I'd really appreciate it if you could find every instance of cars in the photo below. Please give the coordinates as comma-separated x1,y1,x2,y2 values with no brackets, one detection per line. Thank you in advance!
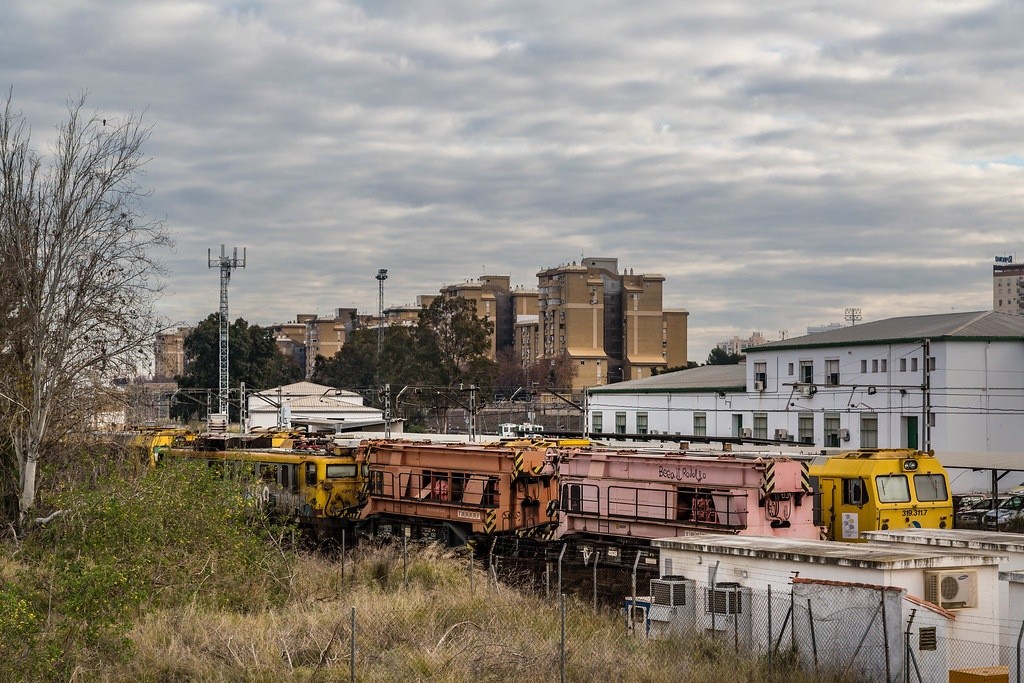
952,481,1024,532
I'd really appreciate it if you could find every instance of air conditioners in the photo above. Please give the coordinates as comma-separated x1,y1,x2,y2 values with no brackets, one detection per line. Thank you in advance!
801,383,810,395
754,381,764,391
837,428,847,439
739,427,753,438
774,429,787,439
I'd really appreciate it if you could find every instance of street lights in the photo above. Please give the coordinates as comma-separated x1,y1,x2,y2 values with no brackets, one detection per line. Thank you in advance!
395,386,409,410
509,387,523,423
374,268,390,384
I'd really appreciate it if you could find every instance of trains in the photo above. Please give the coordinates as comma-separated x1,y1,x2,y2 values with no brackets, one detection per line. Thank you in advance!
43,423,956,542
